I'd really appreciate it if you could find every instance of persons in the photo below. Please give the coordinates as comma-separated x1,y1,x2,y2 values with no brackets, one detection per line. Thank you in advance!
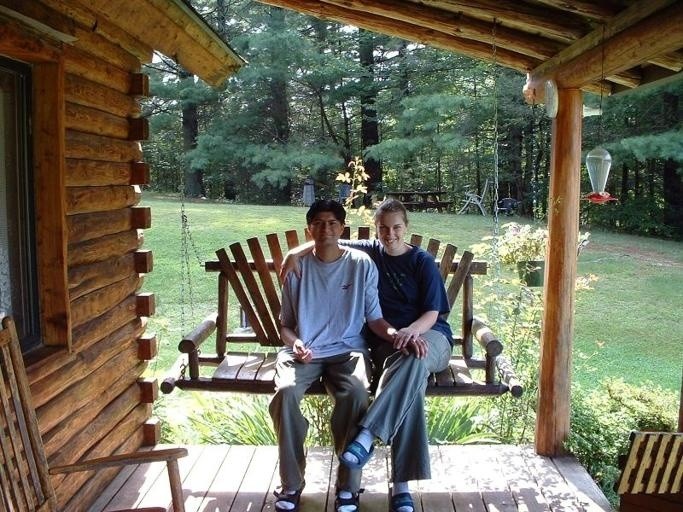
276,197,455,512
268,194,430,512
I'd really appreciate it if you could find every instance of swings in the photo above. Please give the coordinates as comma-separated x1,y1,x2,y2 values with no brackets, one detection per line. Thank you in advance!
161,68,538,396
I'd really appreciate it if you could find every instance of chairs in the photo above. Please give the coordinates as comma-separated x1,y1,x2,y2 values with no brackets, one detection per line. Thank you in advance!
0,316,188,512
457,179,490,217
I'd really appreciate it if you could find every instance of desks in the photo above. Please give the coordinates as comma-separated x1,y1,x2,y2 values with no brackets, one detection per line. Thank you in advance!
385,192,415,212
415,191,448,213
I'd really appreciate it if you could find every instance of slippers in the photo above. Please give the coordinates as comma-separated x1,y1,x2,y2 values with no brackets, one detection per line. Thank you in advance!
339,439,374,469
390,487,415,512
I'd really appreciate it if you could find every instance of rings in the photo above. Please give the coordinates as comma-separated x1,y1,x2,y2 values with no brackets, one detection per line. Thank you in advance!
419,340,424,342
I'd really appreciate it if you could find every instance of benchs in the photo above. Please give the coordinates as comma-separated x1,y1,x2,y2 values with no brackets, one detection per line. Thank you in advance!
159,226,524,398
384,201,454,208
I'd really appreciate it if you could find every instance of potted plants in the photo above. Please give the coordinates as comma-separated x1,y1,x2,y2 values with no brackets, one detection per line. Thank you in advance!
468,221,591,288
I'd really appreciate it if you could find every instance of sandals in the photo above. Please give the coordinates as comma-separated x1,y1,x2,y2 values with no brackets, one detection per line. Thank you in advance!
334,488,364,512
273,480,306,511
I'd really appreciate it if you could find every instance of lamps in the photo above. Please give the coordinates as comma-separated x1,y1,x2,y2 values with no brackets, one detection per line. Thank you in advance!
580,142,619,205
